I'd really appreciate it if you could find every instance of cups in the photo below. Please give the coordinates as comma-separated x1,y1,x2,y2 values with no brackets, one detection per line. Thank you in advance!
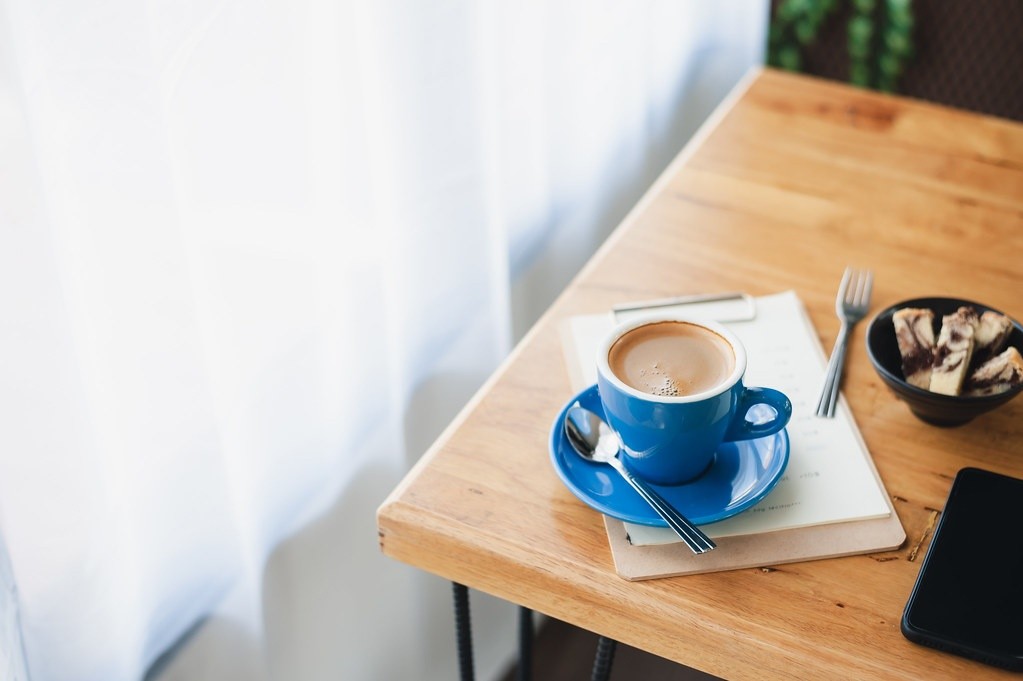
596,314,791,485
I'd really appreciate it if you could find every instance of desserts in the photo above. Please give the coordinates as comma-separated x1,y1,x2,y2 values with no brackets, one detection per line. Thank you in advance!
891,304,1023,397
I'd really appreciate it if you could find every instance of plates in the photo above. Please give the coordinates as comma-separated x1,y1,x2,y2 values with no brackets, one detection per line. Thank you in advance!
548,383,789,528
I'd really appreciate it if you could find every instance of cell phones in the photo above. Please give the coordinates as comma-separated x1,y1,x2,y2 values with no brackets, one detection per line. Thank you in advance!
901,466,1022,672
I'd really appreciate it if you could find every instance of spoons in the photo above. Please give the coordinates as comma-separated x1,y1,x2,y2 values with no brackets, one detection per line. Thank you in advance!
566,408,716,552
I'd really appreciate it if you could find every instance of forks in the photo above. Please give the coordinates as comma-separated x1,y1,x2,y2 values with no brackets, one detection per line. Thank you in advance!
815,269,874,417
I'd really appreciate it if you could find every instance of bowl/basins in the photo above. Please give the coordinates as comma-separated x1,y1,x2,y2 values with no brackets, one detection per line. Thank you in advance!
865,298,1023,429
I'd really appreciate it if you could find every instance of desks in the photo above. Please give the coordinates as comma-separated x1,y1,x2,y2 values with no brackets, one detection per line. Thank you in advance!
374,67,1022,681
